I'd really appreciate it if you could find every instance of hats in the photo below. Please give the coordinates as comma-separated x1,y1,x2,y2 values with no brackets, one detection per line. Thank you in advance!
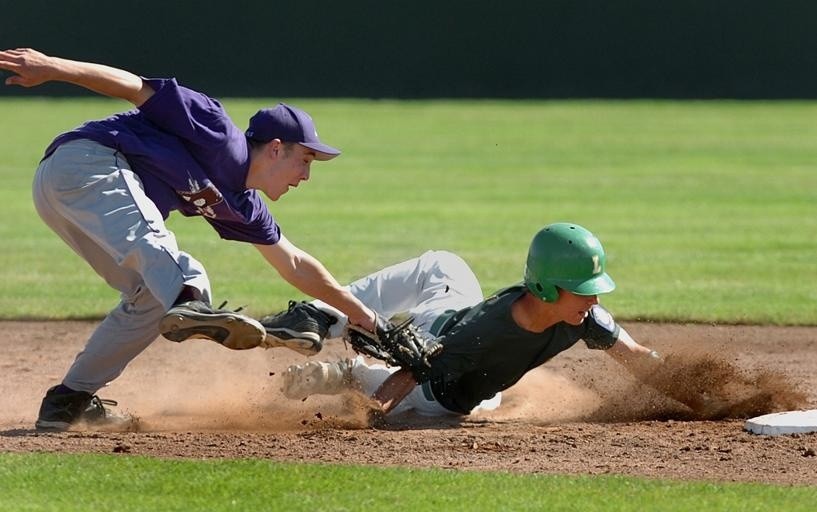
246,103,341,161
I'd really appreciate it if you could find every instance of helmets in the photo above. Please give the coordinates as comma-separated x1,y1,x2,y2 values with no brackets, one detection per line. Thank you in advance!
524,223,616,303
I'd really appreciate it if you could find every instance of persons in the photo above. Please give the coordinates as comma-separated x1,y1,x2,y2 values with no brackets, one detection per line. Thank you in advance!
0,45,443,434
254,218,743,436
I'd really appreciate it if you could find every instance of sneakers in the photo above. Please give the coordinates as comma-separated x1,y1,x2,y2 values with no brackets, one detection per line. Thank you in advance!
159,300,343,399
35,385,121,431
